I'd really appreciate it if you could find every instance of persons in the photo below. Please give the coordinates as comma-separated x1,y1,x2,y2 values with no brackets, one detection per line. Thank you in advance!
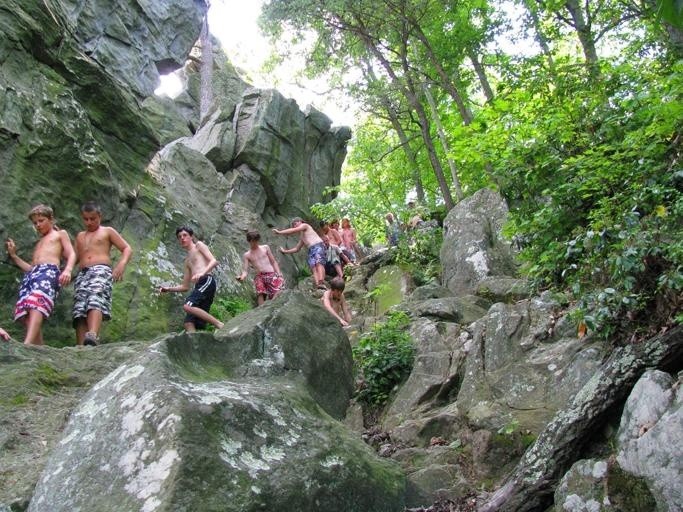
158,226,228,336
321,234,354,278
405,202,430,229
271,216,326,284
339,219,355,250
330,218,350,249
382,213,403,246
70,201,134,348
320,277,352,327
318,220,341,247
4,204,77,342
234,231,284,308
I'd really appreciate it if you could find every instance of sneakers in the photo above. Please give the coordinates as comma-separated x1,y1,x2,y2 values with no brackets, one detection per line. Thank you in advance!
317,280,327,290
83,331,100,346
